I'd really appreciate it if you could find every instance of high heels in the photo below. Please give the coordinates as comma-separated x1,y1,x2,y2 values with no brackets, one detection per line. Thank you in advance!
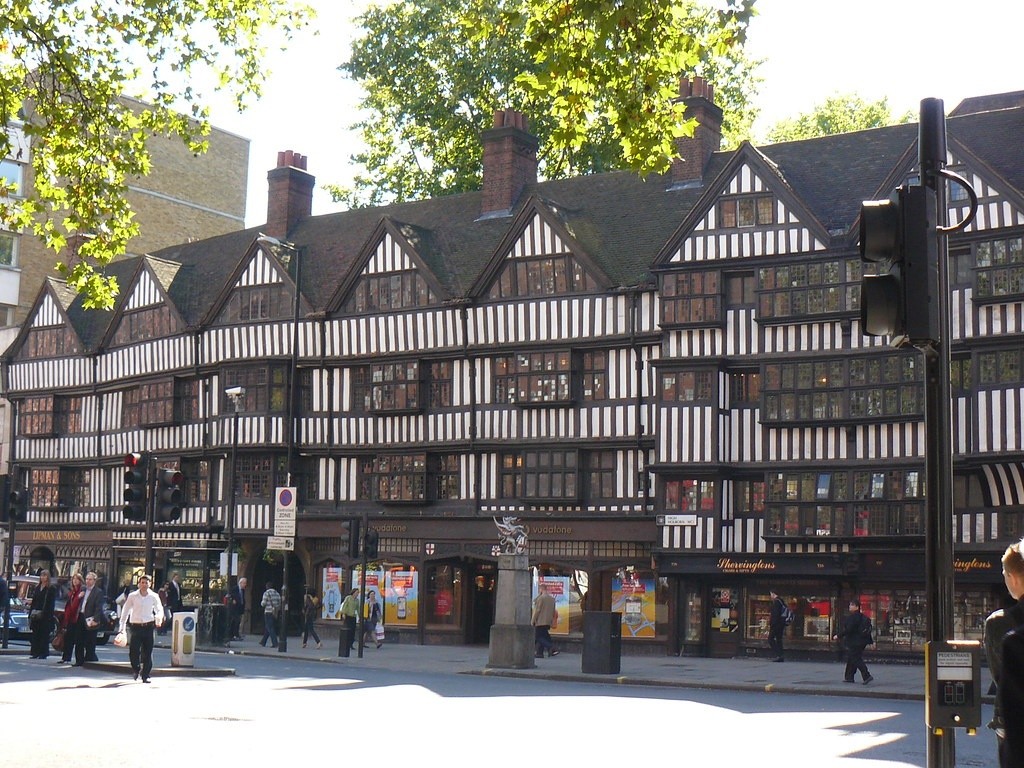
57,659,70,664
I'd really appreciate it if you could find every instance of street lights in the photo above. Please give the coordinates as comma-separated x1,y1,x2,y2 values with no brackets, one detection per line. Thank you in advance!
254,235,302,652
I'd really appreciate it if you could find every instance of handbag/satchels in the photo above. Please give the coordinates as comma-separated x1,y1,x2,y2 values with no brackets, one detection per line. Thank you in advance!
51,626,67,651
85,616,100,627
375,621,386,639
272,606,277,620
335,610,342,620
114,631,128,647
28,609,44,621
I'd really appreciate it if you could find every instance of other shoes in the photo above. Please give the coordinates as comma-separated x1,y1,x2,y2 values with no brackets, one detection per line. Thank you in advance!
362,645,369,648
134,671,139,681
862,675,873,685
376,644,382,649
303,643,307,648
316,641,321,649
72,663,82,667
142,678,151,683
842,679,854,682
30,655,48,659
351,645,356,650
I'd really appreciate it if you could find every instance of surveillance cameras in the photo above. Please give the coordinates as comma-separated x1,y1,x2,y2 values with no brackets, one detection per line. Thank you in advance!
225,386,246,396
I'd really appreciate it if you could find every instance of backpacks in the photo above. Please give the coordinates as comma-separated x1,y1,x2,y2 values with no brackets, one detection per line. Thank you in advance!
775,596,795,626
854,612,872,639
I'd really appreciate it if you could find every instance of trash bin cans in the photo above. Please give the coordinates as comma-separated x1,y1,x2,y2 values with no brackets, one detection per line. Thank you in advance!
339,628,352,658
581,610,624,673
172,611,197,667
200,603,227,644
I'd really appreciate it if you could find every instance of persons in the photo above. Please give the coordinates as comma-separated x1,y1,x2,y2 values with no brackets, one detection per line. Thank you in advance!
767,589,785,662
334,588,383,650
833,599,873,685
259,582,282,649
0,569,183,667
531,584,560,658
984,539,1024,768
302,587,323,649
224,578,249,640
114,575,164,683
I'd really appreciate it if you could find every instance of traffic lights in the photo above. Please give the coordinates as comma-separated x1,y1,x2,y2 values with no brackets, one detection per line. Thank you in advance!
154,469,184,525
123,451,150,522
10,486,27,522
365,531,379,559
860,182,942,347
342,520,359,559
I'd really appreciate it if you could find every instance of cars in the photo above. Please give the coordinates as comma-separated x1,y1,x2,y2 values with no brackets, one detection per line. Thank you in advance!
1,574,120,644
